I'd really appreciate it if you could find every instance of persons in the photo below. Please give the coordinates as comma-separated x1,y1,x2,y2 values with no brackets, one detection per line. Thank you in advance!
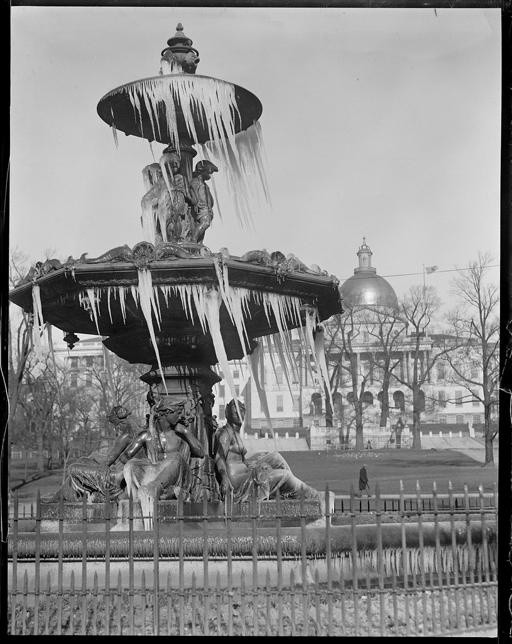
211,396,326,499
122,396,205,501
358,463,371,497
137,152,220,245
67,405,141,501
